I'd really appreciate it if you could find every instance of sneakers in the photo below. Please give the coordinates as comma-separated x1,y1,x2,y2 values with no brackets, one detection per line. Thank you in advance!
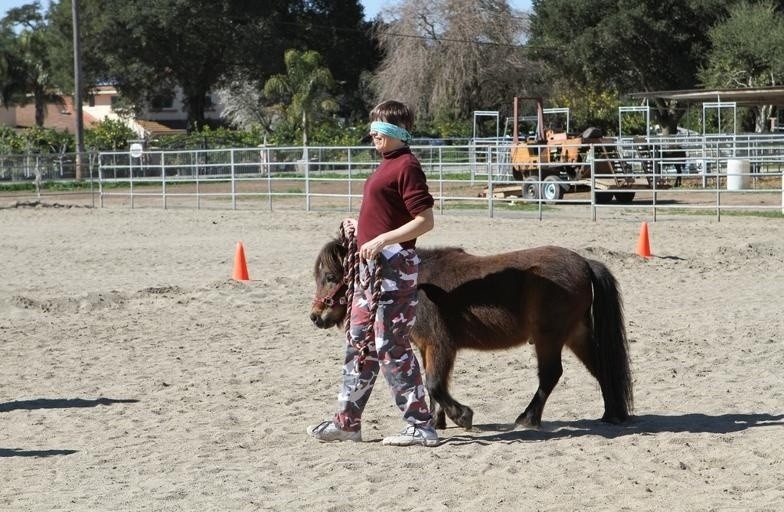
307,422,361,442
382,425,439,446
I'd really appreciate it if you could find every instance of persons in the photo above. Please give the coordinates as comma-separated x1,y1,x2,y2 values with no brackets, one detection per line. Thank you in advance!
307,97,442,447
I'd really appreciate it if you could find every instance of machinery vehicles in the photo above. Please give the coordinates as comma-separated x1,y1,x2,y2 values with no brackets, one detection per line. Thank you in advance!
476,96,671,204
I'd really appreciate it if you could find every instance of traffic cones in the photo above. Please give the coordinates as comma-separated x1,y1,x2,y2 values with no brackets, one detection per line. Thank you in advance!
636,221,650,257
233,241,249,280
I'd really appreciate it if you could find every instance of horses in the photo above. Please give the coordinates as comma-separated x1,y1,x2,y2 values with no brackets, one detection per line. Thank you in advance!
309,232,635,433
651,144,686,187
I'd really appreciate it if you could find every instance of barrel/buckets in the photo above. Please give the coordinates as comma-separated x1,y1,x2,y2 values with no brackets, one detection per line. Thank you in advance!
726,158,751,192
